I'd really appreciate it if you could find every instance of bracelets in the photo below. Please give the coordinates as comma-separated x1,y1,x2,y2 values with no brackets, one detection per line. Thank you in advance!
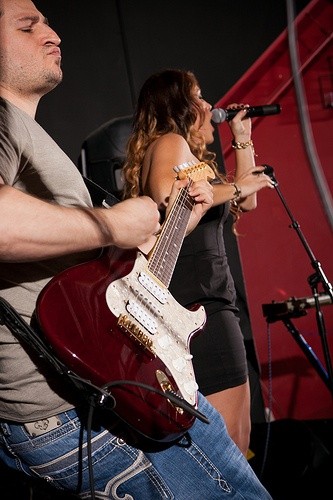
231,140,253,150
231,183,242,198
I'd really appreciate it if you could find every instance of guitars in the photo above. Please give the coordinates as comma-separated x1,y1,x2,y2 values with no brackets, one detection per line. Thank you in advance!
27,158,219,452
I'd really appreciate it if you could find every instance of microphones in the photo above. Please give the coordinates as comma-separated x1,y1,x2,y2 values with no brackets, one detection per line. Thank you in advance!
211,104,282,123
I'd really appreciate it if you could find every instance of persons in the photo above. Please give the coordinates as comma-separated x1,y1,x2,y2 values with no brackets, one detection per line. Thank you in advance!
122,70,278,459
0,0,277,500
